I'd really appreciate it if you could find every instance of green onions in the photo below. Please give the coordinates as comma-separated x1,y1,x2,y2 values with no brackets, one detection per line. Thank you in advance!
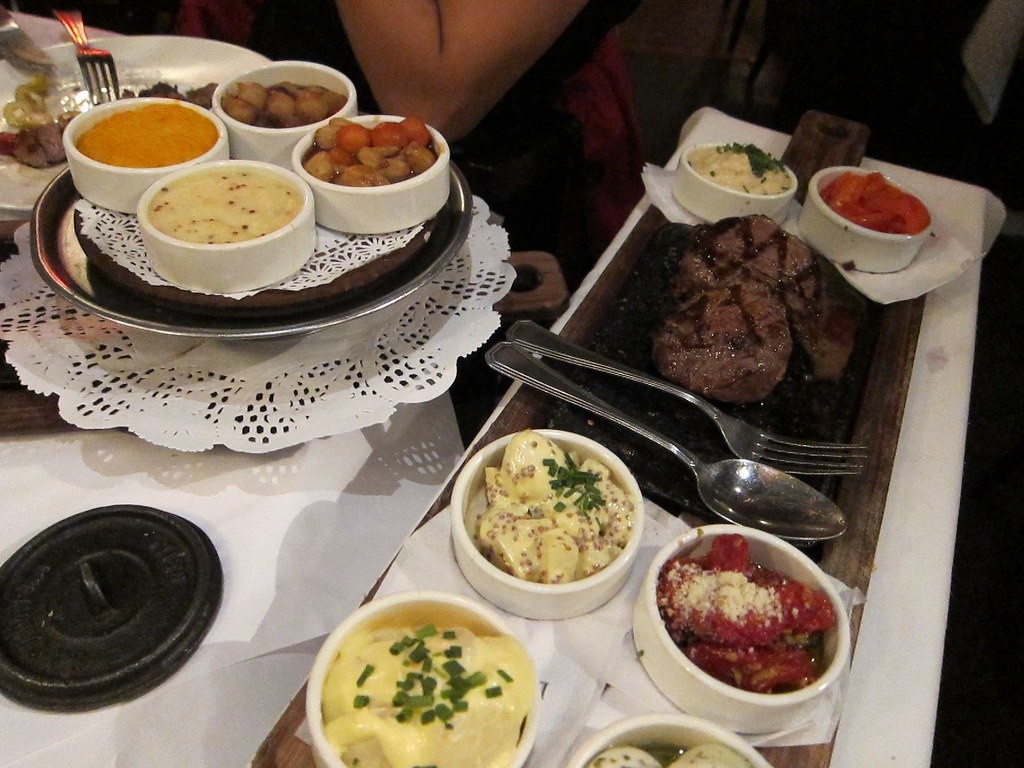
353,624,511,730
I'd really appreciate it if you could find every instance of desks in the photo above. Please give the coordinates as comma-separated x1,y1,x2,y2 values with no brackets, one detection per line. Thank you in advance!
0,10,467,767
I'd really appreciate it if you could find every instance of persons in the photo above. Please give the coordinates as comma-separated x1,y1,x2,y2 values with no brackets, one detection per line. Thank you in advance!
766,1,1024,230
245,1,651,290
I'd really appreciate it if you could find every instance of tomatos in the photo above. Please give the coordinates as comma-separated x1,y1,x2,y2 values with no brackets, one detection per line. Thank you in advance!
821,171,930,235
663,535,835,693
335,117,429,152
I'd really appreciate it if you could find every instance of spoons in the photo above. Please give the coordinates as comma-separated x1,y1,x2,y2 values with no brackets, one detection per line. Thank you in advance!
484,338,847,541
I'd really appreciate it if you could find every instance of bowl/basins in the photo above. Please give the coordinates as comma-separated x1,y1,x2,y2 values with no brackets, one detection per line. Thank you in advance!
564,715,777,766
306,593,540,768
451,427,645,621
211,60,358,165
290,113,451,233
671,141,799,228
799,158,934,271
61,97,231,214
633,523,853,733
136,159,316,295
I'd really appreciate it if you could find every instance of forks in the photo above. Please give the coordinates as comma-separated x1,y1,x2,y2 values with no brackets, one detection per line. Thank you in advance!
51,9,123,103
504,319,871,491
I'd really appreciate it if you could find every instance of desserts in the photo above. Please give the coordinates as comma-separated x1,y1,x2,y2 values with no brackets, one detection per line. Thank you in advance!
146,167,304,245
74,102,219,168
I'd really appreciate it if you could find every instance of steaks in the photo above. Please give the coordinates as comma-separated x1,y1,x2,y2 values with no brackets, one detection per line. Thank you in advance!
648,214,866,405
9,80,220,168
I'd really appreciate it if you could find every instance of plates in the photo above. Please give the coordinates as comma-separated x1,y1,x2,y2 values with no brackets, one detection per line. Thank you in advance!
0,34,297,213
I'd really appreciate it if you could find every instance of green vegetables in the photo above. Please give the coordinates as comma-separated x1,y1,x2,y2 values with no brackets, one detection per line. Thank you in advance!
710,143,790,195
543,453,606,520
